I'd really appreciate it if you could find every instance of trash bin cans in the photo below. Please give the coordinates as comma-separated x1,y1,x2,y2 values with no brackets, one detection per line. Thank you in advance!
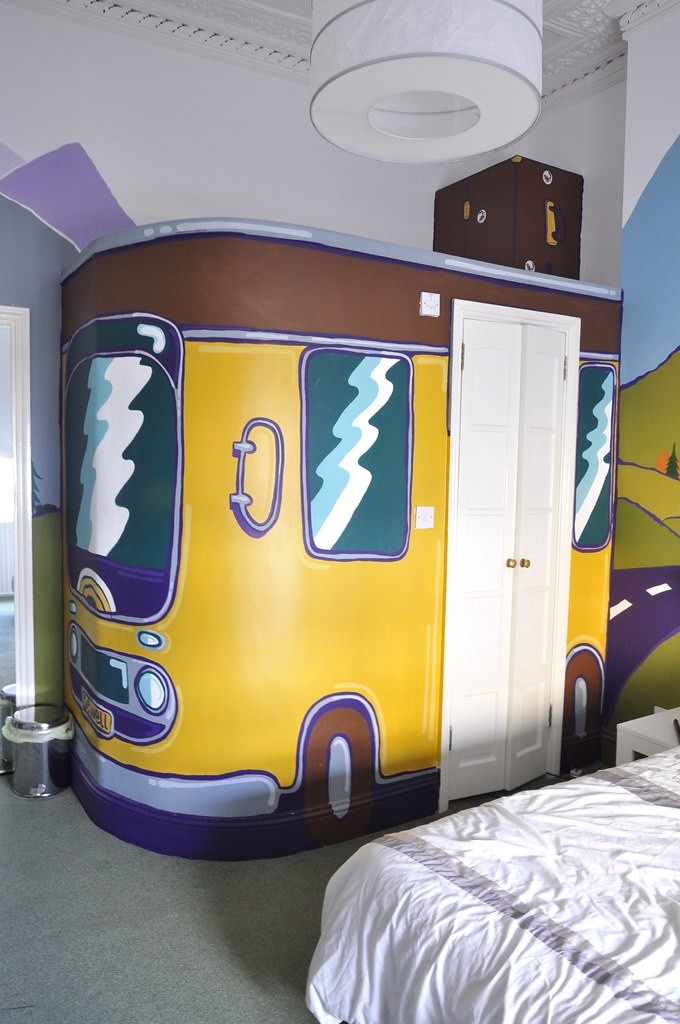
1,705,77,799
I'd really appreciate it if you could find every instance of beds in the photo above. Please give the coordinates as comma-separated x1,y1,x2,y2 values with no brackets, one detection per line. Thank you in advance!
307,747,680,1024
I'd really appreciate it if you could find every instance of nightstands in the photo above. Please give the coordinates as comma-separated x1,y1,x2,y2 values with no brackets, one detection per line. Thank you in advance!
617,707,680,767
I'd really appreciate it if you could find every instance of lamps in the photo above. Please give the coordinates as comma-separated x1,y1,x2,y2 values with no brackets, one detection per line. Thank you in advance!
308,0,543,164
